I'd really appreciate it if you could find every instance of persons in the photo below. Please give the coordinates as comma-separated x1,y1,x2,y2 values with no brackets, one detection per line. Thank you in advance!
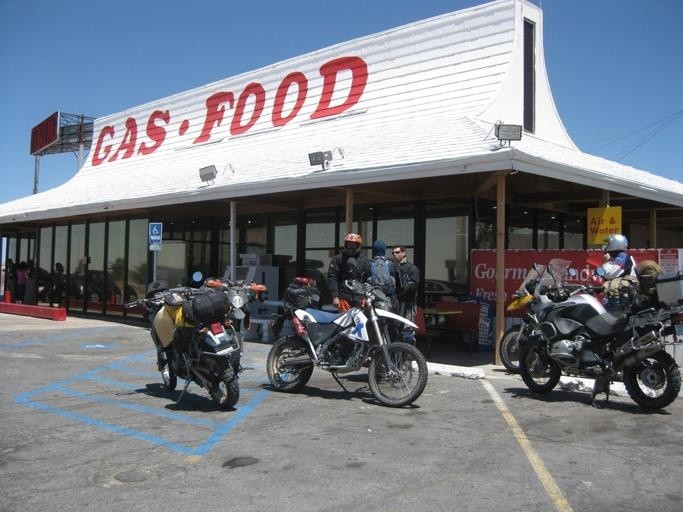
327,233,368,315
595,233,638,312
1,258,16,303
15,262,27,304
368,239,404,377
389,244,421,368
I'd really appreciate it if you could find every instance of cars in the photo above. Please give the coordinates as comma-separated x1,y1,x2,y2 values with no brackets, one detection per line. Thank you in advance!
38,269,147,306
422,277,469,303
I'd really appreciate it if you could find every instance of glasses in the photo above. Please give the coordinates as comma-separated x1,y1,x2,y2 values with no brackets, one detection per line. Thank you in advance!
392,251,401,255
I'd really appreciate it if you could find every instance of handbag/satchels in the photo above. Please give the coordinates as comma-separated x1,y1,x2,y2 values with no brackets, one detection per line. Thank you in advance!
182,290,230,326
601,275,639,307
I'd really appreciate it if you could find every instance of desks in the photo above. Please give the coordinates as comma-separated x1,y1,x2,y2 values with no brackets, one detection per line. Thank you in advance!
263,299,294,309
421,310,463,331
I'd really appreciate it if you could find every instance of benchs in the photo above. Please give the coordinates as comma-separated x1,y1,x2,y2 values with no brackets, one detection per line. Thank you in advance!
428,301,481,353
246,319,275,343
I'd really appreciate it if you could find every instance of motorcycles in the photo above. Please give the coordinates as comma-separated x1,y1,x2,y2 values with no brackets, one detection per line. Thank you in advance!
266,277,429,408
124,270,268,410
498,257,682,410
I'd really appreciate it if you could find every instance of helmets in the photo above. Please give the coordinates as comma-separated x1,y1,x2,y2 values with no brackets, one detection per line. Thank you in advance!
344,234,362,255
603,234,628,253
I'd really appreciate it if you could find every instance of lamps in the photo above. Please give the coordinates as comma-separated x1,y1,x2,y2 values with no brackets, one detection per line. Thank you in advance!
492,122,523,146
199,165,220,184
307,152,336,171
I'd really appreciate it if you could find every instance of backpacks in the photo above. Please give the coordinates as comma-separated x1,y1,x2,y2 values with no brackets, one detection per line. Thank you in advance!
369,258,395,295
